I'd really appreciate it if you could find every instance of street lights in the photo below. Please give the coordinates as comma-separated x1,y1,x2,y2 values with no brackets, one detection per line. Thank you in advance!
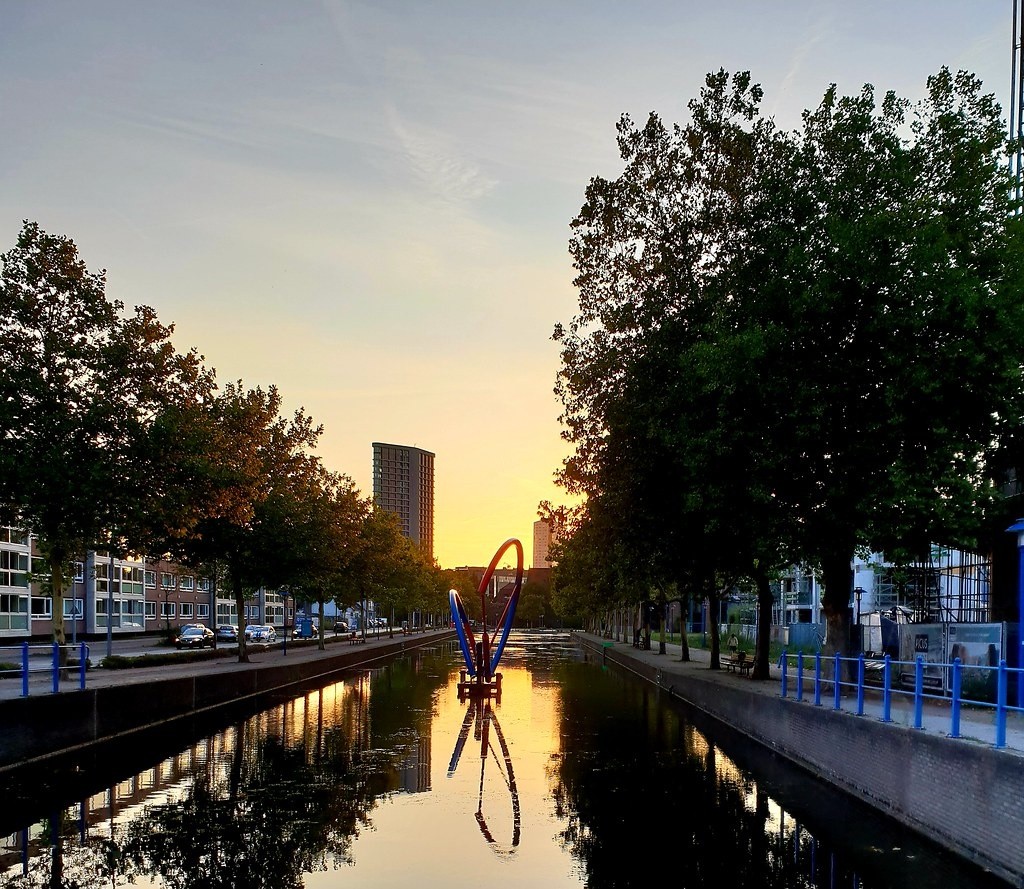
701,601,709,648
670,604,675,641
752,597,761,650
1005,519,1024,711
850,586,868,689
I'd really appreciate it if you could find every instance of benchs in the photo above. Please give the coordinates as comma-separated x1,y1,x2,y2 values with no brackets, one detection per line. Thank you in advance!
349,639,364,645
634,638,647,649
720,653,756,676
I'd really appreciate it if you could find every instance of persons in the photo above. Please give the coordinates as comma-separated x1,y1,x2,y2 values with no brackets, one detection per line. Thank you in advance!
312,621,319,638
635,628,642,648
726,633,738,653
403,625,406,636
382,621,384,629
352,632,358,644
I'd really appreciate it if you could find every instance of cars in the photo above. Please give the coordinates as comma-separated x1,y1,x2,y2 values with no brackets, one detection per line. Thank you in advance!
171,622,205,643
216,625,239,642
333,621,349,633
175,627,215,649
245,624,261,640
292,624,319,638
252,625,277,642
